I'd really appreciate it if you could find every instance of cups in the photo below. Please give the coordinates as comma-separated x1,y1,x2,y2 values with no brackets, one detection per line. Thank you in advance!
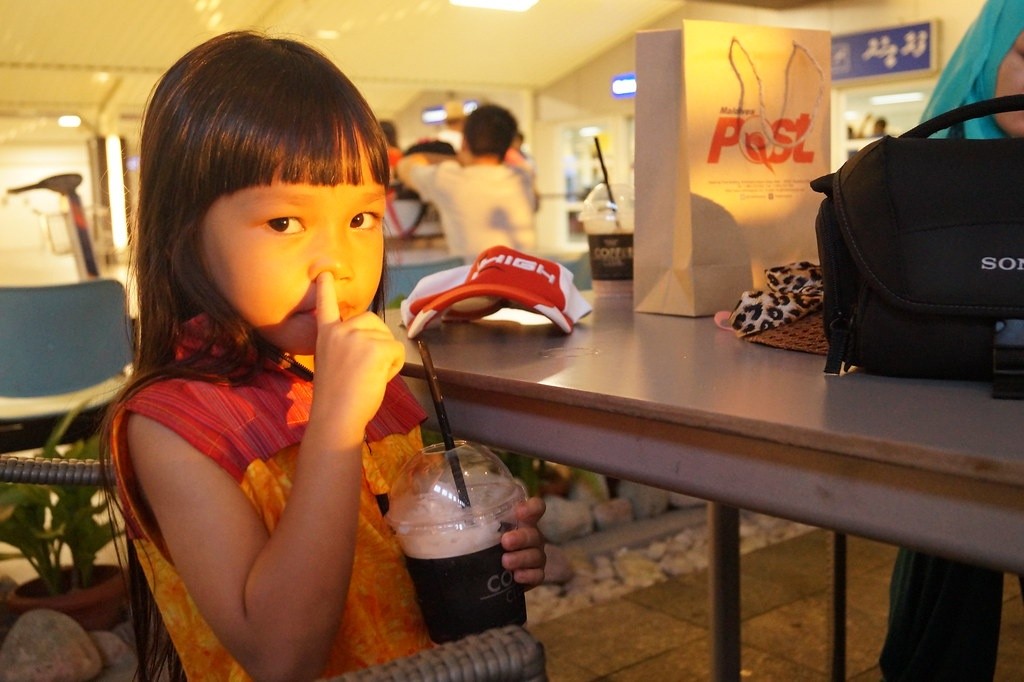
577,178,634,298
382,440,529,648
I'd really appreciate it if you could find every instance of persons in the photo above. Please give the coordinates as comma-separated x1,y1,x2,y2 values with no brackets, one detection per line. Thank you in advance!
104,31,550,682
376,104,540,257
878,0,1024,682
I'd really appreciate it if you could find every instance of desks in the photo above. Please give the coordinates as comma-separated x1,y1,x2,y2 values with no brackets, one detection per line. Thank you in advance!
369,281,1024,682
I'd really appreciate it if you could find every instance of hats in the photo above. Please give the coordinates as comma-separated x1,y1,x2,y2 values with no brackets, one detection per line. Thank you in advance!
730,261,830,356
402,244,592,339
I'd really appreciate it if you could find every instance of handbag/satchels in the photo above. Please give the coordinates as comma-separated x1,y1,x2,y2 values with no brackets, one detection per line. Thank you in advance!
635,20,831,317
810,93,1024,400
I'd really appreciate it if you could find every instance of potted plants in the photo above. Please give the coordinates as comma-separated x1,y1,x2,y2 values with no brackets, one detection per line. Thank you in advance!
0,379,129,631
